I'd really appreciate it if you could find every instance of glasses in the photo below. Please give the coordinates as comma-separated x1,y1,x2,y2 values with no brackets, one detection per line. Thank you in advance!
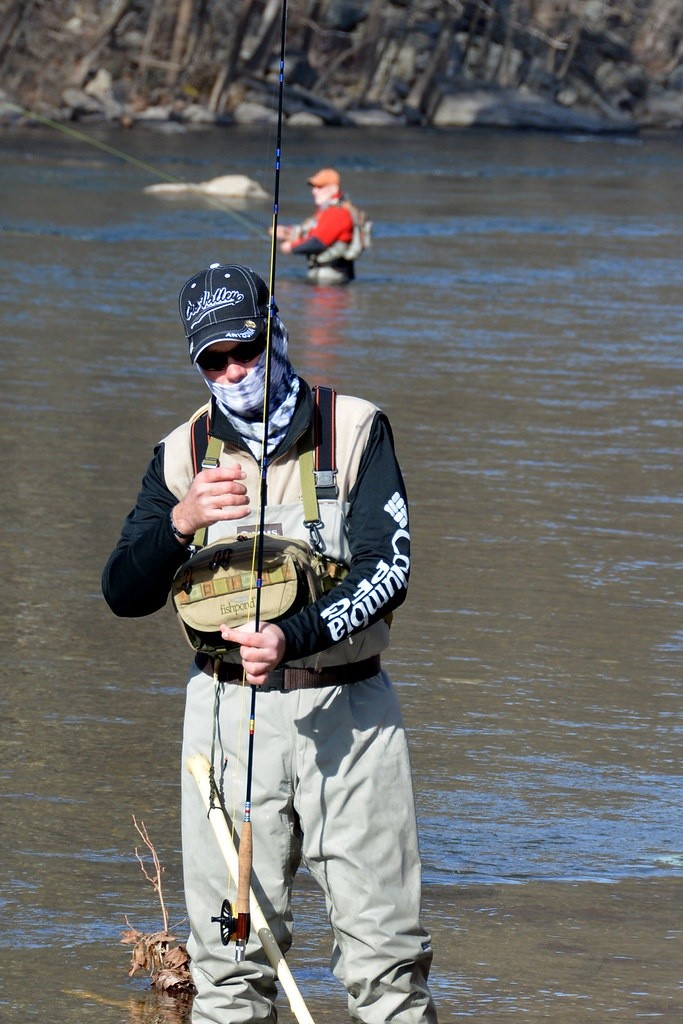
196,332,266,371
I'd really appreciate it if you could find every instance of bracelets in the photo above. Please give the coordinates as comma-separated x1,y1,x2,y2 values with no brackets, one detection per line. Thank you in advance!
170,512,195,539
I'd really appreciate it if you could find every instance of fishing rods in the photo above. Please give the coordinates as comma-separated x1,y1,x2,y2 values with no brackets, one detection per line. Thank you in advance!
0,95,283,242
223,0,291,971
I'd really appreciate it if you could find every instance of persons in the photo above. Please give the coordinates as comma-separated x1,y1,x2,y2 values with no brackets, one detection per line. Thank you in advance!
101,263,439,1024
269,169,355,284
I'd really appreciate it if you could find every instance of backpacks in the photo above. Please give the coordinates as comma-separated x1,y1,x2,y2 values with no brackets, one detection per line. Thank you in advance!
338,200,375,261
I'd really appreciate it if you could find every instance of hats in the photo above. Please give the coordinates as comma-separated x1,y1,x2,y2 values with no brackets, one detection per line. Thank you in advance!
178,263,279,366
307,167,341,187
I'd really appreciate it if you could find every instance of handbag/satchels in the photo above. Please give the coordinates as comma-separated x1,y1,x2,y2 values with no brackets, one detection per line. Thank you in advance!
171,436,331,657
